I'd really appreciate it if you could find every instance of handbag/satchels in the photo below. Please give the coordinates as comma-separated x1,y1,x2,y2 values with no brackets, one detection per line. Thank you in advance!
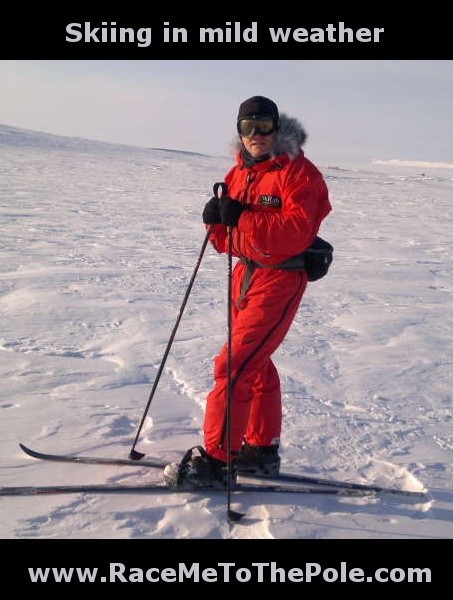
240,236,333,282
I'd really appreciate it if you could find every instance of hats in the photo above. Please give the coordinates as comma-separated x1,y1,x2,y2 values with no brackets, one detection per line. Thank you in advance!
237,96,279,127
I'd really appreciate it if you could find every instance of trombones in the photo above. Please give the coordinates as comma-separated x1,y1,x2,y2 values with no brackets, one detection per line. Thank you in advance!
0,443,422,496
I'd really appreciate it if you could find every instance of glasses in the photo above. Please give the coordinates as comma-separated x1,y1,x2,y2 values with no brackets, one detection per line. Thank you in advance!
237,115,275,136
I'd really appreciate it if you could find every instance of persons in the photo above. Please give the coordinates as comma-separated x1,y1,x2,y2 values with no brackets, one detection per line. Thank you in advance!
163,94,333,491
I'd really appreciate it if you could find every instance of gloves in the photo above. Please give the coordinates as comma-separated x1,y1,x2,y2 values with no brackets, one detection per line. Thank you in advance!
203,197,222,224
219,198,242,226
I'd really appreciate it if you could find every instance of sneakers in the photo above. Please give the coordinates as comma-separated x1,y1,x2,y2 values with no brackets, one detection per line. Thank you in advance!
238,444,280,480
163,445,239,490
